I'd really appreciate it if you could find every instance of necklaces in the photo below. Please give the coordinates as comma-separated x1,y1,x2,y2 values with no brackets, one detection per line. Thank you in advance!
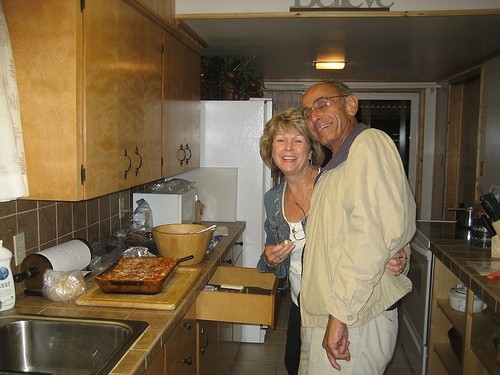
286,166,315,234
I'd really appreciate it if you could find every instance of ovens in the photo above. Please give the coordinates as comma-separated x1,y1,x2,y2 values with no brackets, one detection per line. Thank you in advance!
398,234,433,375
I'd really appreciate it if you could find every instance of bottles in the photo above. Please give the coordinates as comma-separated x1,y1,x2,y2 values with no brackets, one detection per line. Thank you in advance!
127,198,153,231
0,240,15,312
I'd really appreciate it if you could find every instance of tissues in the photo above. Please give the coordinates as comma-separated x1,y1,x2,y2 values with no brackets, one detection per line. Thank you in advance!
491,217,500,259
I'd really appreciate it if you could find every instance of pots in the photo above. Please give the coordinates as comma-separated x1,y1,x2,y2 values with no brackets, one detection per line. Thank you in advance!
447,207,475,228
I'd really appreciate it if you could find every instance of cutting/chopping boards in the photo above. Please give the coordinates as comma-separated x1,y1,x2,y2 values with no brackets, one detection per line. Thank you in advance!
75,267,202,311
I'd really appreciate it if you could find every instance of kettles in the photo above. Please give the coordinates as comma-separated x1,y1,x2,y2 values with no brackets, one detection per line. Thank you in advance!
449,284,488,312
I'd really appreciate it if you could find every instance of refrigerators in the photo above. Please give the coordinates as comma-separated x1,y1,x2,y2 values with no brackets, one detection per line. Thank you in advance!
198,97,275,344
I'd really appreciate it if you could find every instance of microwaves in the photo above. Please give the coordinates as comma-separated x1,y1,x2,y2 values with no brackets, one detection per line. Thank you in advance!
133,187,197,226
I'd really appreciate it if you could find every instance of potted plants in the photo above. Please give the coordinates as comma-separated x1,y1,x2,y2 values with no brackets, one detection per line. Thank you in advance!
218,54,267,100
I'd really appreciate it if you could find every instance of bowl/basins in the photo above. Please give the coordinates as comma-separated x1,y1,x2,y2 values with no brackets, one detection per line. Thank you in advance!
447,327,463,364
151,224,213,266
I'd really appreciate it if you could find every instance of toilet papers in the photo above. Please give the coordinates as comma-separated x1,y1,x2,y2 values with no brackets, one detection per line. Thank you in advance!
20,239,94,292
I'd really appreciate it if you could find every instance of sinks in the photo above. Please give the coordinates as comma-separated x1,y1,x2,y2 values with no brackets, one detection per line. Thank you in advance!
0,310,151,375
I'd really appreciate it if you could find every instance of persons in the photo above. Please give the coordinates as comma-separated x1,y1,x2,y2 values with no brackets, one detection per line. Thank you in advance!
256,106,407,375
296,80,417,375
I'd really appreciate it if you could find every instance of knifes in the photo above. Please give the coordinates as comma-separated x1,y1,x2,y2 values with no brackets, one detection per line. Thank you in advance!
479,192,500,236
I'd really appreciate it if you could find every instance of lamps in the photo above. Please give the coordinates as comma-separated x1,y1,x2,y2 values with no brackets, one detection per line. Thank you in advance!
311,50,351,71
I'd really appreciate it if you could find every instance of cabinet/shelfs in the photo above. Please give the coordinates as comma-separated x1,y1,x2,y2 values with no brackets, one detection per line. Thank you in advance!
136,221,280,375
398,235,500,374
0,0,209,203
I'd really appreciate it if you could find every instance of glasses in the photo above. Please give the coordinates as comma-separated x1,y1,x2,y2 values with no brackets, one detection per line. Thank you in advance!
302,94,349,121
273,201,307,246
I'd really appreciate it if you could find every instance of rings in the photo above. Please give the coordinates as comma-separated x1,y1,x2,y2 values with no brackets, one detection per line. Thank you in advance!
279,257,284,262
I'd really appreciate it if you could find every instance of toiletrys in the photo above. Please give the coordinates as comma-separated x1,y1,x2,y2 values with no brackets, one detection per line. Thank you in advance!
0,239,16,312
